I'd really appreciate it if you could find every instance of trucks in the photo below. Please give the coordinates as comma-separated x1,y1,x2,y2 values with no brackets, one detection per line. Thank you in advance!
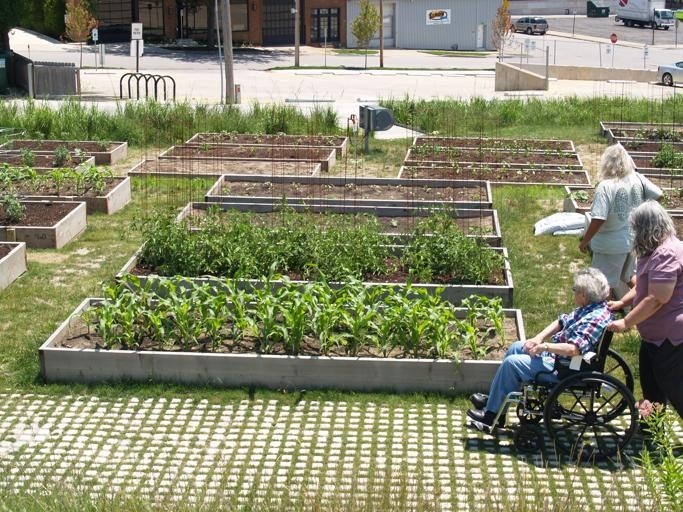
615,0,675,31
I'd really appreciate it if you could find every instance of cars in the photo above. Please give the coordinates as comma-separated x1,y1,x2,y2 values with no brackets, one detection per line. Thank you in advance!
657,60,683,86
510,16,549,35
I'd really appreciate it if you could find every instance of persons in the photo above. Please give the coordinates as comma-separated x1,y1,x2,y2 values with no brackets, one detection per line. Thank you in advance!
462,265,613,427
605,196,683,438
578,141,664,319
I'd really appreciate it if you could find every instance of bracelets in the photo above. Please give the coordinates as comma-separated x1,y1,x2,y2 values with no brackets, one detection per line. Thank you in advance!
542,341,548,354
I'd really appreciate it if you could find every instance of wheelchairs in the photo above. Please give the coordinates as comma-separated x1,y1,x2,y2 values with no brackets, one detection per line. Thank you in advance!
464,308,639,461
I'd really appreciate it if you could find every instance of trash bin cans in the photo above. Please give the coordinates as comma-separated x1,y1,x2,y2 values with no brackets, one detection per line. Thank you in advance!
565,9,569,15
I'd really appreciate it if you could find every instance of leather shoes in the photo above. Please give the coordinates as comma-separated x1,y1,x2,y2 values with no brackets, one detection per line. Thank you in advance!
466,393,506,427
